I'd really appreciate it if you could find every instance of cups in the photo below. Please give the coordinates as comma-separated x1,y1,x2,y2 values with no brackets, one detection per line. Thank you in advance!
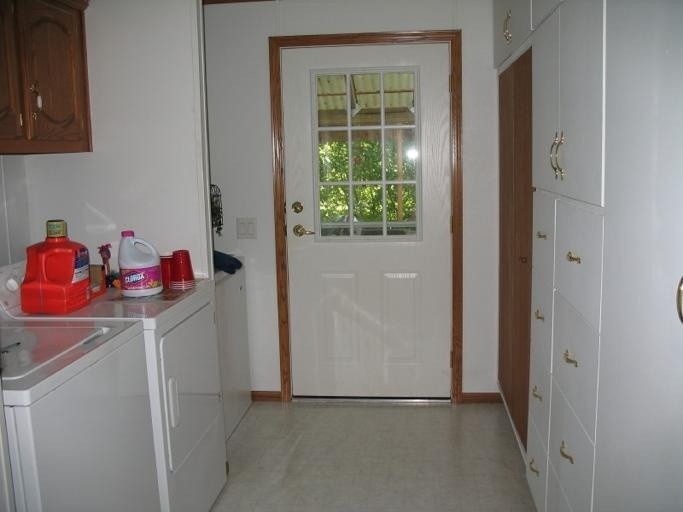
159,249,195,290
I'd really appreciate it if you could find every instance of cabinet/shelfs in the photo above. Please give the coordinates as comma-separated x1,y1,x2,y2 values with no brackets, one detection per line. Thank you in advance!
0,0,91,153
492,1,562,66
531,0,607,208
525,192,605,512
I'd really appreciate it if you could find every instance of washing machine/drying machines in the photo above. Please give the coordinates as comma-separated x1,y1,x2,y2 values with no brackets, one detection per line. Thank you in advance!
0,260,229,512
1,302,161,512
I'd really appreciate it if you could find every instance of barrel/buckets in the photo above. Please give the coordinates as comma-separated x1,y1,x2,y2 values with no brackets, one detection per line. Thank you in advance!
117,232,164,296
19,221,92,314
